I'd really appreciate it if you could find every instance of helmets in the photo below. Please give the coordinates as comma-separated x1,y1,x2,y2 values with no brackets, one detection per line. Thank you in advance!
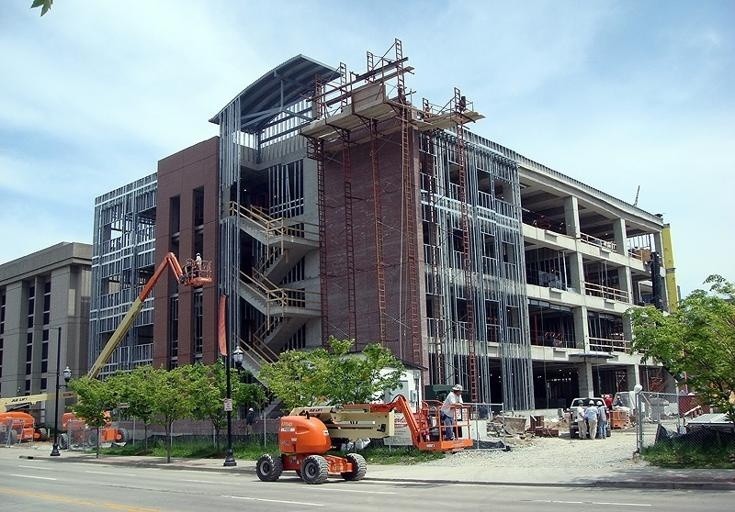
577,399,602,406
452,383,463,391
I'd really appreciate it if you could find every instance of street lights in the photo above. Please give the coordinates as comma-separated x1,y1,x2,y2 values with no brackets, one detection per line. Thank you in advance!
224,294,245,467
49,324,72,456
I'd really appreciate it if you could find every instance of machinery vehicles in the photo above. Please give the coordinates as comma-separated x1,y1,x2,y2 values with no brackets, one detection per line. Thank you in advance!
0,390,78,443
256,393,474,483
59,250,214,450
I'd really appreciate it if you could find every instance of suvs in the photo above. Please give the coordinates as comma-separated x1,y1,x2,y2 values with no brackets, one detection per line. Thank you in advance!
566,397,612,437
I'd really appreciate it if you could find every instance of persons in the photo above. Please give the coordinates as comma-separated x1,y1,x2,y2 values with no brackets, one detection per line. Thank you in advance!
605,395,611,407
585,400,598,439
577,399,587,439
194,252,203,277
246,408,258,435
597,401,608,439
440,383,465,441
613,396,622,406
